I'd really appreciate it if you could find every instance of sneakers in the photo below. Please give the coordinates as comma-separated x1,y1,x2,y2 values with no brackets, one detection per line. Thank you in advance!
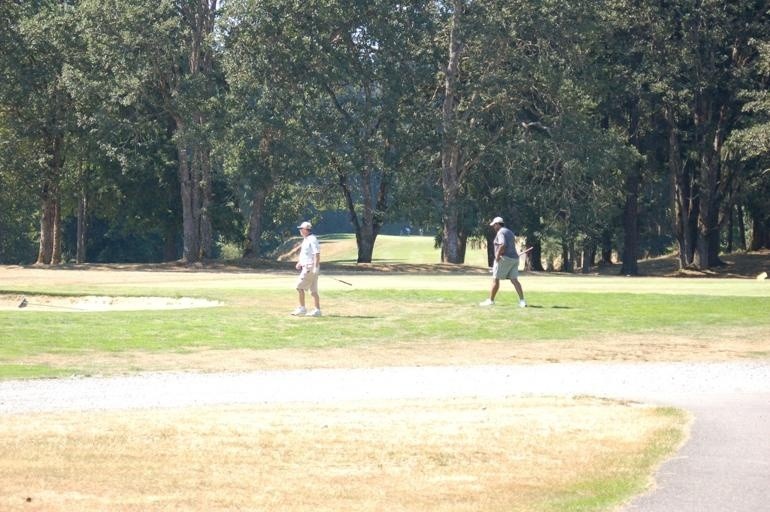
480,298,495,306
305,307,321,317
291,306,306,315
519,299,527,308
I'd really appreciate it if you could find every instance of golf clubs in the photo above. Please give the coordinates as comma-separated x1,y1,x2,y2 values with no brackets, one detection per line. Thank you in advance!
307,265,352,286
489,246,534,273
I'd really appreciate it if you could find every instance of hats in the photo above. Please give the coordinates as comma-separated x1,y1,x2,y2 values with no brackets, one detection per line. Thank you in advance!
489,216,504,227
296,222,312,231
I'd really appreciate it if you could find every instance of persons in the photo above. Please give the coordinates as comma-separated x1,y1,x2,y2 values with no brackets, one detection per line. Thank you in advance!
479,215,528,308
294,220,321,317
